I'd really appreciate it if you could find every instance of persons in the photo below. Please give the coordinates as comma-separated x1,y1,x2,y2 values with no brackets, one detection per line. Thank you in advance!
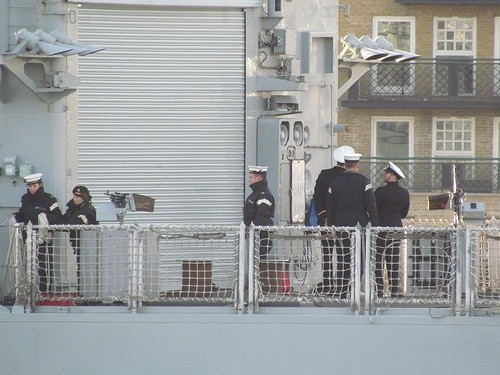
313,145,357,295
243,165,275,260
326,154,380,300
10,172,62,295
47,184,100,296
373,161,410,299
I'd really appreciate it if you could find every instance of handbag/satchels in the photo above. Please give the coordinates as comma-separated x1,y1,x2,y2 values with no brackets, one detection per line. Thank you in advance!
306,199,319,227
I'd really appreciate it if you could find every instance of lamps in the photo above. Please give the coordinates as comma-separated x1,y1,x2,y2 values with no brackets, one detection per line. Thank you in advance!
10,28,106,57
339,33,422,64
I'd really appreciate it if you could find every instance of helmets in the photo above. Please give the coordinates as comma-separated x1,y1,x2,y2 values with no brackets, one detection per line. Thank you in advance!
72,186,92,201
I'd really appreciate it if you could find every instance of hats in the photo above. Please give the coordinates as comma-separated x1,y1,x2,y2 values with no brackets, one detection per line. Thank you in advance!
343,153,362,163
383,161,406,180
23,173,43,185
248,166,269,174
334,146,355,166
